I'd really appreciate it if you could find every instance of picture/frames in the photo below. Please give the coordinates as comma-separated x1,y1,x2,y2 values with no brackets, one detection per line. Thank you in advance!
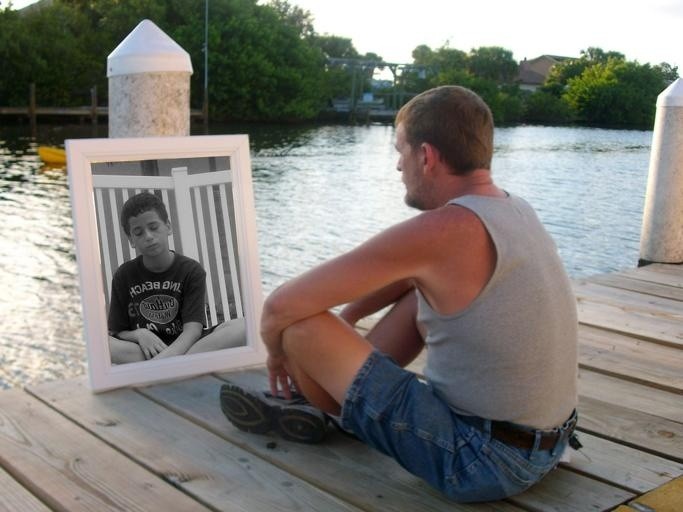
59,132,269,397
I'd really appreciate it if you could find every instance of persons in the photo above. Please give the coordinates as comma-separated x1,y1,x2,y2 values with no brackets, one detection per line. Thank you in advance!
219,85,582,504
108,192,246,365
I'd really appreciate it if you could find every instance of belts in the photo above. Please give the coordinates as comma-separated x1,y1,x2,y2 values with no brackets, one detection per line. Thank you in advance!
453,412,561,453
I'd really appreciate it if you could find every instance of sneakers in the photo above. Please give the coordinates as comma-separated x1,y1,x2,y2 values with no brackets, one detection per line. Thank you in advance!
218,382,337,445
